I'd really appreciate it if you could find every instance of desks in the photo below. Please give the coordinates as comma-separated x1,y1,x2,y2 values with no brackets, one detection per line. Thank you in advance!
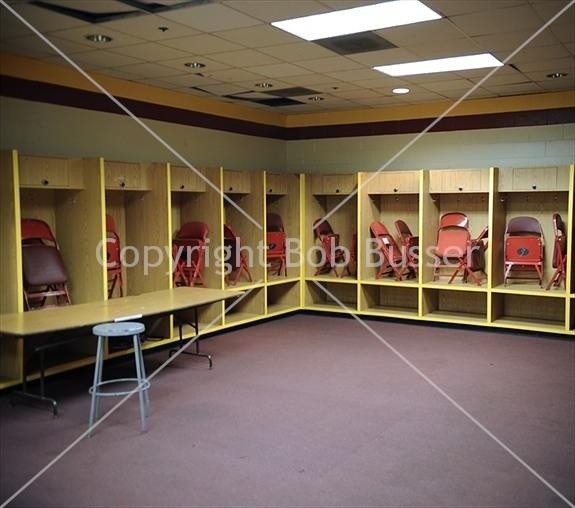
0,286,245,416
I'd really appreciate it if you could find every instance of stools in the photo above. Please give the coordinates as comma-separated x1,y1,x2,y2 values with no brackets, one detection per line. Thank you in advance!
89,323,152,438
